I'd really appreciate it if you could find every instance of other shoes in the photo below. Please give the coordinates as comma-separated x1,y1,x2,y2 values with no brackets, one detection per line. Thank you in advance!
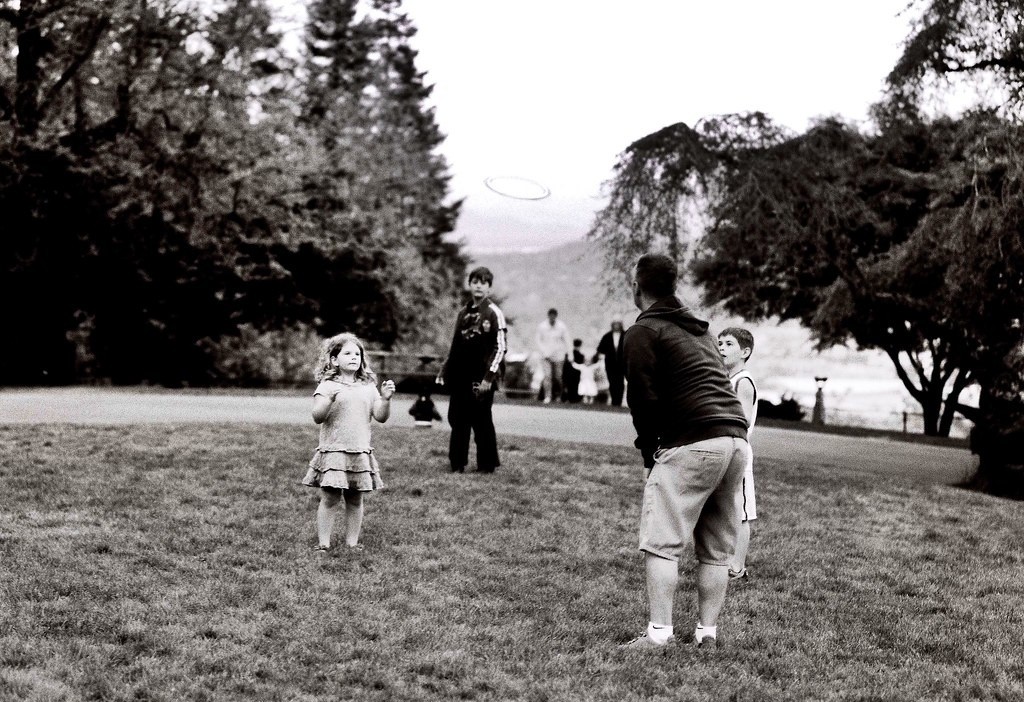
446,463,464,474
727,563,749,582
475,465,495,474
682,630,718,650
617,633,678,654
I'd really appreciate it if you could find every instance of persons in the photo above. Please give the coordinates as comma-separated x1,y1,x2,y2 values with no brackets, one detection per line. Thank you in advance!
435,267,509,473
302,333,396,552
528,308,624,407
616,254,750,651
715,327,758,581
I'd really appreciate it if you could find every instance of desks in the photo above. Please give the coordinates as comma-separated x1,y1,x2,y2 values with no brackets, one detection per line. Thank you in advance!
365,349,441,395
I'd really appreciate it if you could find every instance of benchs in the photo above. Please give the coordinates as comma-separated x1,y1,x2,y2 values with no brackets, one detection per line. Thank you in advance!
371,369,437,381
